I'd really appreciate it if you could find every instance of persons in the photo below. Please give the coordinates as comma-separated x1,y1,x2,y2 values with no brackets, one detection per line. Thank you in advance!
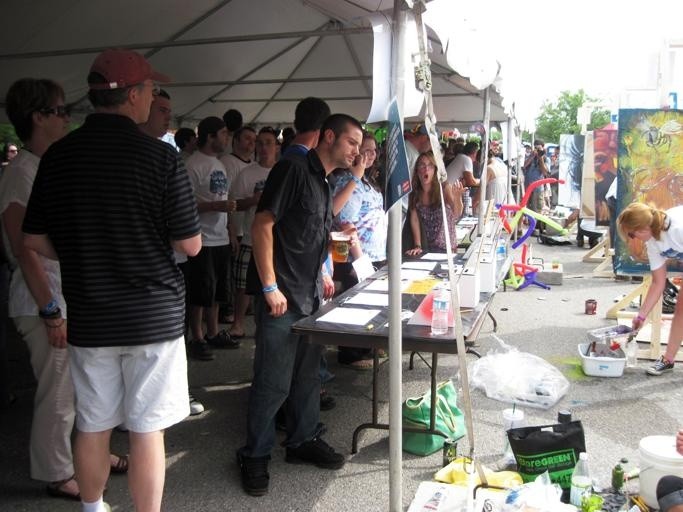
174,127,198,160
0,141,20,168
222,109,243,133
218,125,257,187
400,124,442,230
233,113,365,498
224,126,281,339
404,151,469,256
333,134,389,263
470,138,520,216
656,431,683,512
138,87,173,138
615,202,683,377
21,48,207,511
276,97,368,438
443,138,480,186
1,77,130,502
182,115,263,362
520,138,559,213
378,139,387,193
564,209,609,248
279,126,295,162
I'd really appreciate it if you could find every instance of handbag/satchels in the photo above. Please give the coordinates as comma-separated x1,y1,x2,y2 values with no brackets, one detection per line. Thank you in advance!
401,379,465,457
506,412,587,506
537,234,571,245
661,278,679,313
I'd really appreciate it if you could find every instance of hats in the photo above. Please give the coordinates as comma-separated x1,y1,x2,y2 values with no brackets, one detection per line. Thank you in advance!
260,127,281,137
417,125,440,137
88,50,171,90
491,142,499,146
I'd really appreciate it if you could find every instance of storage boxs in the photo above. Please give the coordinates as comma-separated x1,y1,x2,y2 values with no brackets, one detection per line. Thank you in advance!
578,342,625,377
588,324,635,348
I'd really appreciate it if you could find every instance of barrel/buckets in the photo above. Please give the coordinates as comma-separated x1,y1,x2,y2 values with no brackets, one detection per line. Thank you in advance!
637,433,683,509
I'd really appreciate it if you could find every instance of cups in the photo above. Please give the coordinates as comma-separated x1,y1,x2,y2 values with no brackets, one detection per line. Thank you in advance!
581,494,604,512
501,408,525,456
330,231,349,263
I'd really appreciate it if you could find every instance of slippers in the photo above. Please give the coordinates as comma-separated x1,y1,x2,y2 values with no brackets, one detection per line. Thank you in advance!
364,346,388,358
341,360,372,370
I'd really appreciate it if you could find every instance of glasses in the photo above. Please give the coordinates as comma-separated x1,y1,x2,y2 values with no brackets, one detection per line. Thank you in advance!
628,228,638,239
136,80,161,96
415,162,434,171
41,105,72,119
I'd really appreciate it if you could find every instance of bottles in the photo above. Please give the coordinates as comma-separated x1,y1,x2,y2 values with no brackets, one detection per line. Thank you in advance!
495,240,507,270
569,452,592,508
248,344,255,380
431,285,450,334
627,338,638,368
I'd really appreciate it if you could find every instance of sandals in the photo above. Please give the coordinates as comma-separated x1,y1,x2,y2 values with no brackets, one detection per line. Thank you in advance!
110,450,130,473
45,475,108,500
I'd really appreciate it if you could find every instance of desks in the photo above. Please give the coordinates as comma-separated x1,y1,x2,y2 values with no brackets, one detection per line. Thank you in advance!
290,251,512,455
438,212,522,333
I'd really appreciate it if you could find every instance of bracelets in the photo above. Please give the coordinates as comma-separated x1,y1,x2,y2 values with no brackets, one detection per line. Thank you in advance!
236,197,244,212
351,175,359,184
262,284,279,293
43,317,65,329
637,315,646,322
414,244,422,248
38,298,62,319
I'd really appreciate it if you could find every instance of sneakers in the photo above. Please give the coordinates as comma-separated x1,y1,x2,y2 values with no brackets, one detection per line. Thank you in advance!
590,242,597,248
189,389,205,415
646,355,674,376
320,391,337,411
577,239,584,246
285,436,345,471
221,311,235,324
275,421,327,438
237,447,270,496
189,339,215,360
203,329,239,349
